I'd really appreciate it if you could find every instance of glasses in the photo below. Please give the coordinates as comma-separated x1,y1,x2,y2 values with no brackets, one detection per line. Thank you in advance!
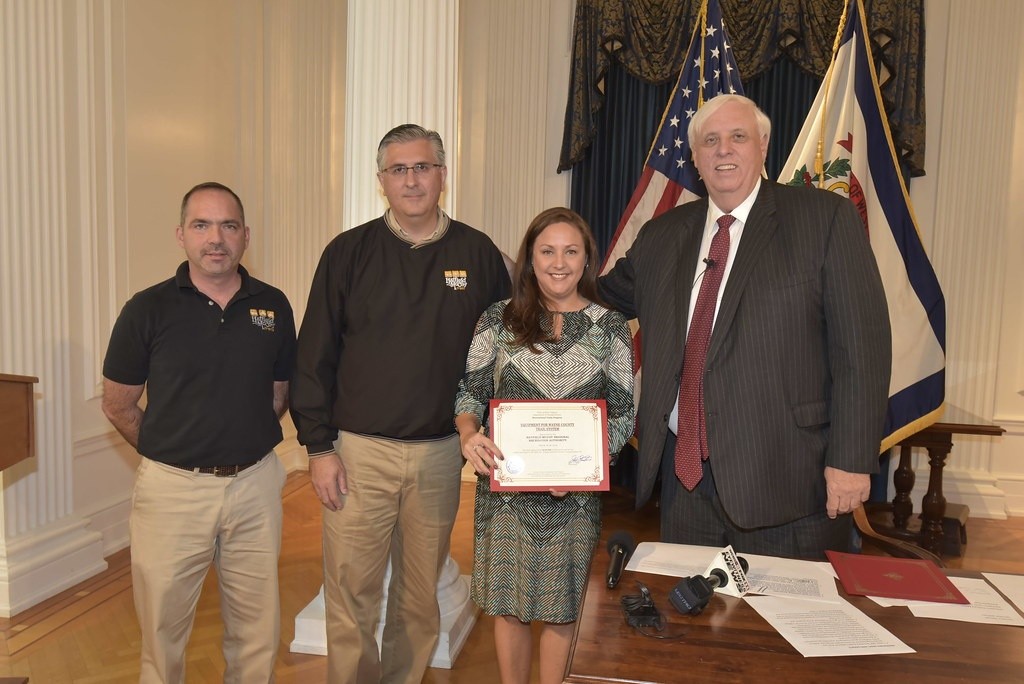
381,162,442,176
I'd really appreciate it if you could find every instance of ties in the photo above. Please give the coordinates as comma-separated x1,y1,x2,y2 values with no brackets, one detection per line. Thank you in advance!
675,215,738,491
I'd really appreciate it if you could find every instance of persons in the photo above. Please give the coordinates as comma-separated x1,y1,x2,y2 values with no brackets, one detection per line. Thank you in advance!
101,181,296,684
501,94,892,560
454,207,635,684
287,123,513,684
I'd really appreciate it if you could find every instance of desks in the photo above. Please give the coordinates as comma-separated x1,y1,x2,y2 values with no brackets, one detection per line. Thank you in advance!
561,539,1024,684
870,400,1004,552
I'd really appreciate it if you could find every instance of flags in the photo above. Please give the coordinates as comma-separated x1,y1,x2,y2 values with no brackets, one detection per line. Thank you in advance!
596,0,770,452
777,0,946,458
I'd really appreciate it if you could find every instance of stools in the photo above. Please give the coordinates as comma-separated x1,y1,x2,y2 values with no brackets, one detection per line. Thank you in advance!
918,504,969,557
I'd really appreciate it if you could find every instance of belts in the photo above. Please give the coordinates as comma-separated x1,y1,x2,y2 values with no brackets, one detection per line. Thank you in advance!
164,456,256,477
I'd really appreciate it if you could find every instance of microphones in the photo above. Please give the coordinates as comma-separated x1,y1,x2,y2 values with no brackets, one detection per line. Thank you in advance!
606,532,634,589
668,545,749,616
706,259,714,269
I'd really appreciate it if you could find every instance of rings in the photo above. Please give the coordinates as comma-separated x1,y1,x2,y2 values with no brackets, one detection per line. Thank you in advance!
473,444,482,451
